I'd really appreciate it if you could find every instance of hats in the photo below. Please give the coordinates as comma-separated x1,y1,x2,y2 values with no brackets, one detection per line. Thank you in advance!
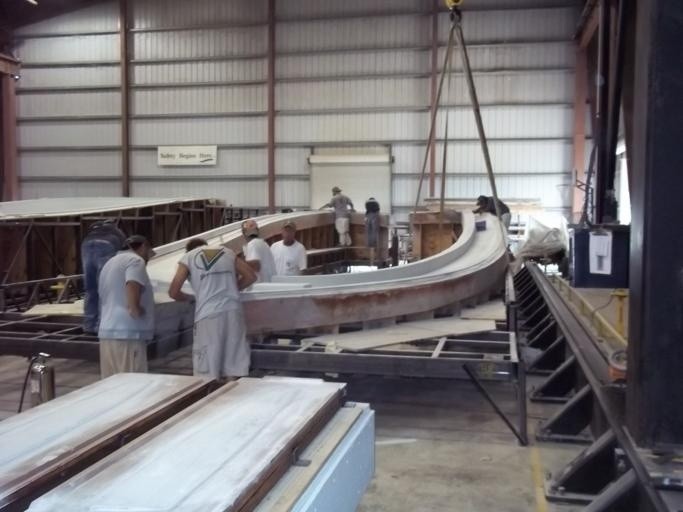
241,220,258,237
476,195,487,206
283,220,296,229
122,236,156,258
333,187,341,192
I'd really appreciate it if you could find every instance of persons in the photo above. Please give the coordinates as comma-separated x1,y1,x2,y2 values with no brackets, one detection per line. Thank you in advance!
168,238,257,381
473,196,512,231
365,195,380,245
97,235,157,379
326,185,354,246
270,221,308,345
240,220,275,283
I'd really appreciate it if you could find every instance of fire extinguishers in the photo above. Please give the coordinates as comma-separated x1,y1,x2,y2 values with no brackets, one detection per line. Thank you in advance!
18,353,54,413
